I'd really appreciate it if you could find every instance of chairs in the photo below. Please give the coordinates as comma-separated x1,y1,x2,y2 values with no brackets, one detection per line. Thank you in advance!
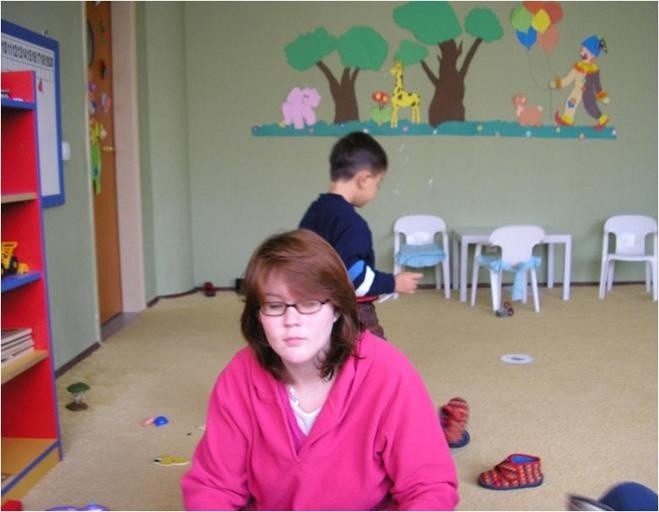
392,214,452,299
470,224,540,313
598,213,657,302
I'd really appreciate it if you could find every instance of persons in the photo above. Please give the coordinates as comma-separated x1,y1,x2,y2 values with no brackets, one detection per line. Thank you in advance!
296,130,424,342
178,226,461,511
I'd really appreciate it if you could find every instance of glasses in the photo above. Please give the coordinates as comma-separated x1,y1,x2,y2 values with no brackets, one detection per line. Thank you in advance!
260,299,329,316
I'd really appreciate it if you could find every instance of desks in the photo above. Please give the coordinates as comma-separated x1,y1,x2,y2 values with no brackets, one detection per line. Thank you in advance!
452,228,573,304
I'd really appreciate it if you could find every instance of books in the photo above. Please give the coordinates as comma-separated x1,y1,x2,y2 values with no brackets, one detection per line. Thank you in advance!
1,327,35,368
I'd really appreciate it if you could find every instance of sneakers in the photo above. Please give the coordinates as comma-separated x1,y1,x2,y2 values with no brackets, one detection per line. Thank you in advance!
440,398,469,447
479,454,542,490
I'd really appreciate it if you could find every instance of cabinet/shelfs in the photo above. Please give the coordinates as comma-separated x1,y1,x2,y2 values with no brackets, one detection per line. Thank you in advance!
1,68,64,507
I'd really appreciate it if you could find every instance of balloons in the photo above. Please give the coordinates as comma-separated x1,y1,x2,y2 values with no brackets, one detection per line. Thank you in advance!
510,1,563,50
